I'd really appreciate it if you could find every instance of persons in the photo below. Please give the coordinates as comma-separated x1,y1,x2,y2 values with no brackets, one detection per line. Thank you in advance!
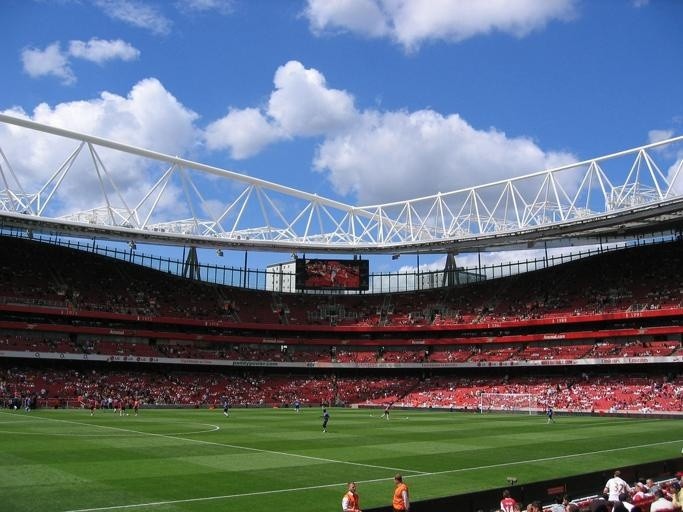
544,403,556,426
320,405,330,433
341,480,361,511
379,399,395,424
390,472,411,511
492,460,683,511
0,234,682,417
